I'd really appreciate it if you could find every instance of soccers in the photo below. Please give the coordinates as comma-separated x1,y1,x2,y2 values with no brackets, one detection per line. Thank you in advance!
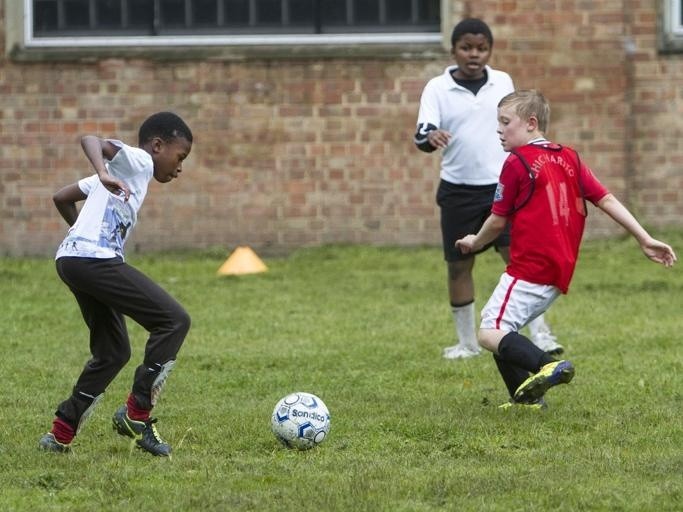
269,390,329,449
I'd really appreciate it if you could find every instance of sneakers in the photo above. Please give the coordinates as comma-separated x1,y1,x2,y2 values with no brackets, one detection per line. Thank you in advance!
36,431,74,455
531,334,565,355
497,397,548,411
440,343,482,360
512,360,576,406
111,402,172,457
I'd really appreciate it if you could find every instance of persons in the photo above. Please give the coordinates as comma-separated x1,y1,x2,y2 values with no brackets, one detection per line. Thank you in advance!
412,17,566,358
35,111,193,458
454,88,676,412
